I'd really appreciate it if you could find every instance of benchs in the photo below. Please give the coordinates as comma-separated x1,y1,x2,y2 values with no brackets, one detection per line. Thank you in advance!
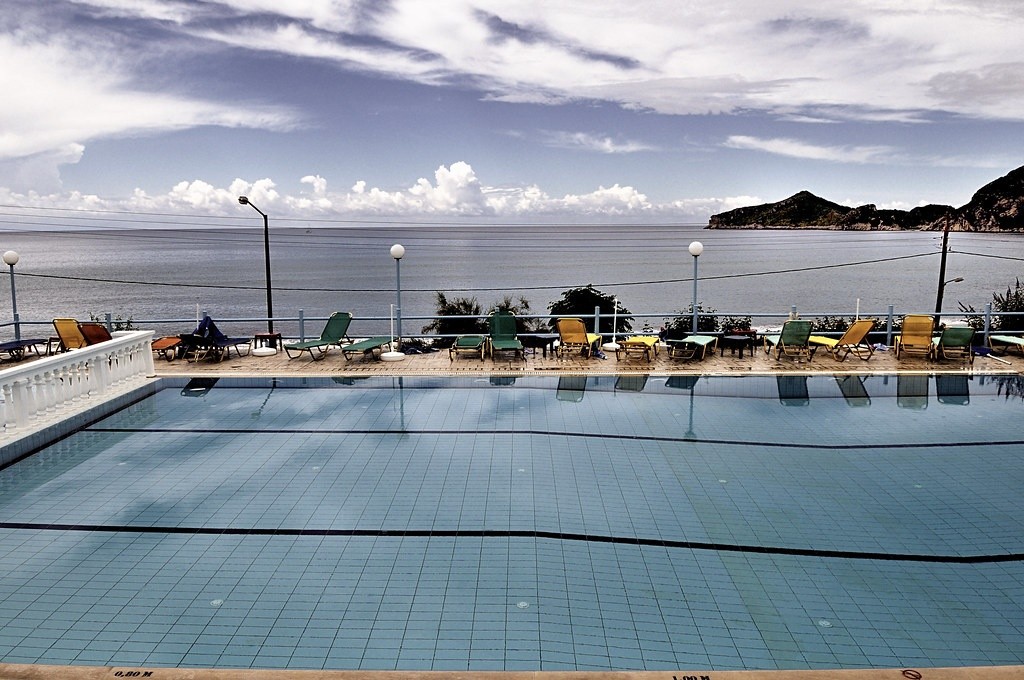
807,319,877,362
989,334,1024,356
764,319,813,361
894,314,935,362
666,335,718,361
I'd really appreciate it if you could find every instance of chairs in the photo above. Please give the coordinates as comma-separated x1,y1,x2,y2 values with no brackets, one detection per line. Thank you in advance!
615,376,648,392
332,377,355,385
53,318,113,355
0,339,48,365
556,376,587,402
339,336,401,362
449,335,488,362
776,376,809,407
936,376,969,405
897,376,928,409
151,316,253,363
555,318,602,360
490,377,516,386
489,311,526,362
834,376,871,407
283,312,354,361
615,336,660,363
180,377,220,397
932,326,976,365
665,376,699,389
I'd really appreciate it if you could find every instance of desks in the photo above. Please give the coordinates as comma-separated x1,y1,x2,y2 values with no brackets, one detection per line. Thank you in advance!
518,335,560,358
732,328,757,350
255,332,282,351
721,336,753,359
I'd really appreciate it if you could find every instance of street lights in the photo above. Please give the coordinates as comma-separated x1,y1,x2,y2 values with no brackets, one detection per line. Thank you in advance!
250,378,276,420
3,251,21,339
238,196,273,334
688,241,704,336
934,276,964,329
684,386,697,443
390,244,405,345
397,376,410,441
